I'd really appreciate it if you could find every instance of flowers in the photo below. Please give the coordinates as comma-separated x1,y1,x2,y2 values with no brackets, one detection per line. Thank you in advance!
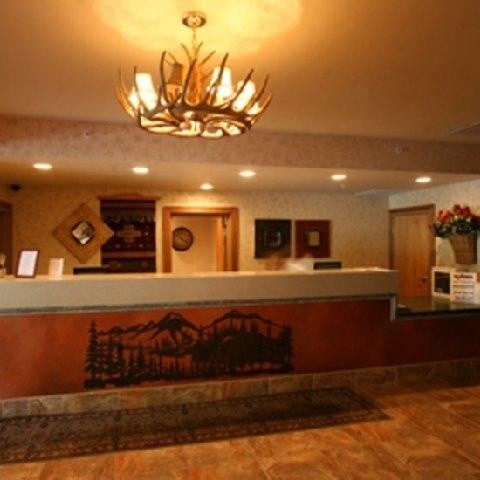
432,203,480,236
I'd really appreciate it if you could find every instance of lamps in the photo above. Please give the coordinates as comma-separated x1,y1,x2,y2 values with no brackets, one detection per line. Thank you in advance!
112,41,273,138
